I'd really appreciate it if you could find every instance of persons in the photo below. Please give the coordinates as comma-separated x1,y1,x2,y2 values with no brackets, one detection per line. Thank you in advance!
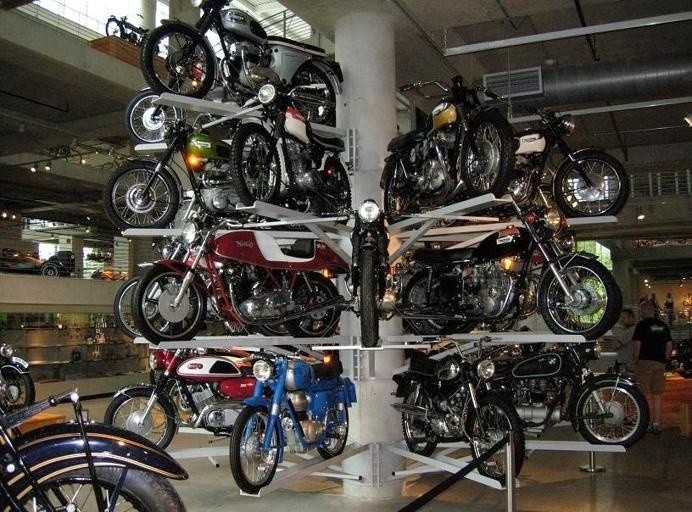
611,309,638,424
638,293,677,326
629,300,674,434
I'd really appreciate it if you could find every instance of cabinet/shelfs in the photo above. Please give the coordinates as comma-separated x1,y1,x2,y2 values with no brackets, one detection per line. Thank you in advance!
89,36,169,80
0,324,151,383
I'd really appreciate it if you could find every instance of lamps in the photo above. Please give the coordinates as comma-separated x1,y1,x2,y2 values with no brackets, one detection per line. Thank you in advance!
30,145,99,173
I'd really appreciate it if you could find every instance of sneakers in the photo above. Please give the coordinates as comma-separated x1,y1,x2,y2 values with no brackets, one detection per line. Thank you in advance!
647,422,662,435
